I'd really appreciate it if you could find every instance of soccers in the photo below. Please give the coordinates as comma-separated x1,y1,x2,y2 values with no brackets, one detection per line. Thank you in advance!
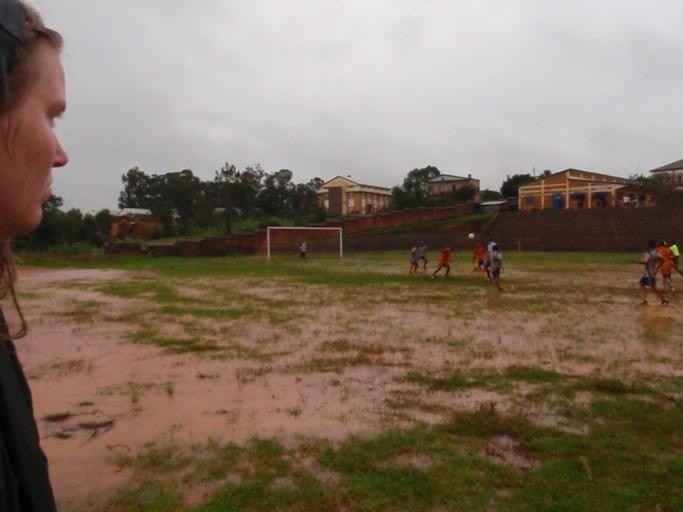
469,233,474,238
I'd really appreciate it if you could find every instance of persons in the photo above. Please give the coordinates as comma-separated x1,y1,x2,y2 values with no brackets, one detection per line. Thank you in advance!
636,237,683,307
615,192,642,209
299,240,307,261
1,0,71,508
407,233,505,293
